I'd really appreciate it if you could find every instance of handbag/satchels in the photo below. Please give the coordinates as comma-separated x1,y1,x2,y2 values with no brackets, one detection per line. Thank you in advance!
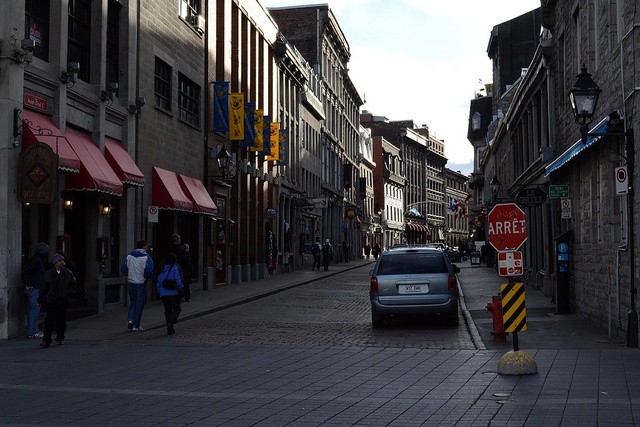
329,253,334,261
162,263,177,290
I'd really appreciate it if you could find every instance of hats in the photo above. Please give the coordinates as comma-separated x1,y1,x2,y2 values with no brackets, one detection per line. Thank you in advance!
49,253,65,264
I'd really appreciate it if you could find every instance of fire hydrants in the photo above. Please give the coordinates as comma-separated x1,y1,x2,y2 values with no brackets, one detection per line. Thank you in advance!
485,296,509,342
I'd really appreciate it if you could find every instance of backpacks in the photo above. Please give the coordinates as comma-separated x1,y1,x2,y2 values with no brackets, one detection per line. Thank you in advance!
311,244,320,256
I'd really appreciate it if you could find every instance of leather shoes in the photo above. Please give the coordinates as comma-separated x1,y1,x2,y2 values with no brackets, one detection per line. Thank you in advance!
167,322,175,334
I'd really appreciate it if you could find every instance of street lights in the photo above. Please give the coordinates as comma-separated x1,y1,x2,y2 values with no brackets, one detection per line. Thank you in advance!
490,175,500,203
203,143,231,291
567,63,640,346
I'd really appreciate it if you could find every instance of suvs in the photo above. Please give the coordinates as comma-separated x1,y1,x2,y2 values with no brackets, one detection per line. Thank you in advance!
369,248,460,327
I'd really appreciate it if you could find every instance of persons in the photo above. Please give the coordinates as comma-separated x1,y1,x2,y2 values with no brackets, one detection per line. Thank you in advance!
169,233,185,299
181,243,193,302
365,244,370,259
121,239,154,330
459,241,465,261
25,242,49,338
372,243,379,258
157,253,184,334
312,237,323,271
40,253,77,347
322,239,333,270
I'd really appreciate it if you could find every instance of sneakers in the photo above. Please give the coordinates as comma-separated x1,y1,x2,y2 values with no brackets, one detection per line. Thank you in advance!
39,331,43,334
128,320,132,328
40,340,48,347
133,325,144,330
29,333,44,338
58,338,65,344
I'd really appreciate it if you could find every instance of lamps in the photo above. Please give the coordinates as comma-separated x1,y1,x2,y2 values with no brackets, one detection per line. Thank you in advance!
568,61,627,143
490,174,515,203
207,142,231,181
